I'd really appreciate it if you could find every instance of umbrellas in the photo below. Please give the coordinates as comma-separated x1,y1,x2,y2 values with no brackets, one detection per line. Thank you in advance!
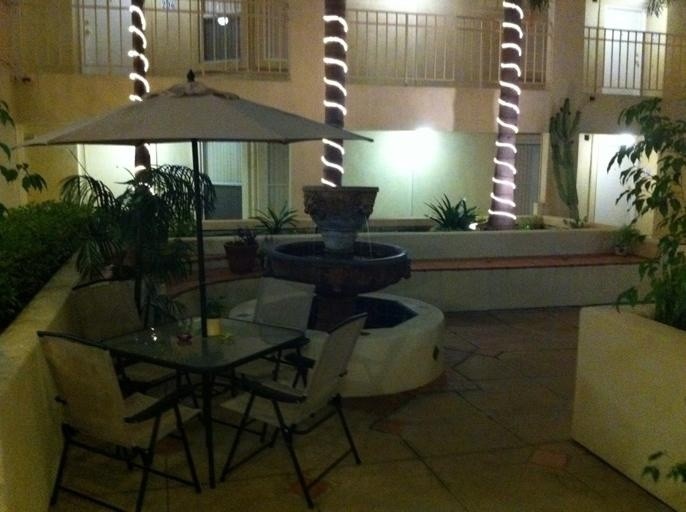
10,66,376,489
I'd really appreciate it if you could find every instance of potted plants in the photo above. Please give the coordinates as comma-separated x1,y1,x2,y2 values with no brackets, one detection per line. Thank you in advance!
223,227,260,274
571,94,686,512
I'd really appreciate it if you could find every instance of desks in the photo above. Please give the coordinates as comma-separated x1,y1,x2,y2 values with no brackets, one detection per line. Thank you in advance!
102,316,305,486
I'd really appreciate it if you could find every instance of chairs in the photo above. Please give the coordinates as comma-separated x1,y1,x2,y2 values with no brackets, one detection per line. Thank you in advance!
202,279,315,443
218,310,368,511
37,329,200,512
70,279,199,408
